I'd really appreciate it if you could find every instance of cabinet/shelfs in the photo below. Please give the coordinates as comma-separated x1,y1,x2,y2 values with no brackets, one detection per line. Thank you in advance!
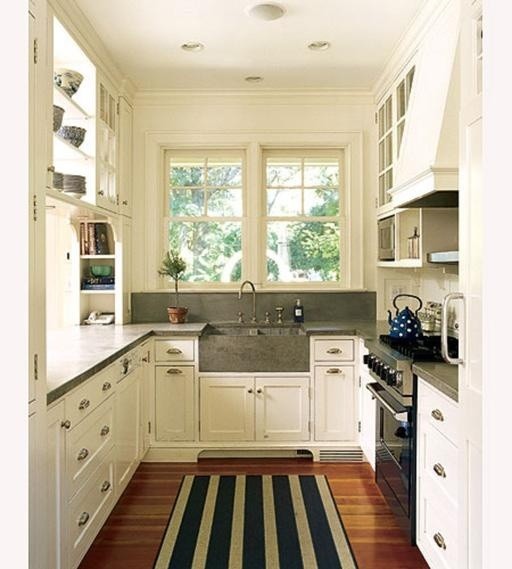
64,362,117,559
46,399,68,568
152,334,199,448
310,335,361,445
116,338,150,488
375,0,460,267
46,1,133,325
357,334,377,472
415,375,459,569
442,0,483,569
200,372,312,445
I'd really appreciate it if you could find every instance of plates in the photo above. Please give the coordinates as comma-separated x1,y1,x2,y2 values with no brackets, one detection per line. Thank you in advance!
53,174,88,197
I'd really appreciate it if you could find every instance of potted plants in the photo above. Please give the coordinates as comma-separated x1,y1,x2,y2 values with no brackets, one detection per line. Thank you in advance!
157,252,188,323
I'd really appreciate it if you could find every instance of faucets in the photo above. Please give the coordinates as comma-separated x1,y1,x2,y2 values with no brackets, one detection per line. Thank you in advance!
237,279,258,322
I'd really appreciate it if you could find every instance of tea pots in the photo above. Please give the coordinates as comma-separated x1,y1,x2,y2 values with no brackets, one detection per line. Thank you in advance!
388,294,423,340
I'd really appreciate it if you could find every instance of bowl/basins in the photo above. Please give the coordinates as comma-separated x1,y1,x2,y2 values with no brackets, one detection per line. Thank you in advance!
88,263,111,277
52,68,87,147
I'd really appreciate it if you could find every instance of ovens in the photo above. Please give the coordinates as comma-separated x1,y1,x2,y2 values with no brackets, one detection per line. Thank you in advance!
363,382,415,545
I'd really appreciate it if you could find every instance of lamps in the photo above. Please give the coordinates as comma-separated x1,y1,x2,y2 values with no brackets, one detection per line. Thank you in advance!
245,2,287,23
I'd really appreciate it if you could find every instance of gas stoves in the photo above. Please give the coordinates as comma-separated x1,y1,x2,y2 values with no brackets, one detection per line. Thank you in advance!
363,335,457,406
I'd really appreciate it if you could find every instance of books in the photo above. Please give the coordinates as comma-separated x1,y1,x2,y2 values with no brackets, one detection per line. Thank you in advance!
81,224,109,255
82,276,114,290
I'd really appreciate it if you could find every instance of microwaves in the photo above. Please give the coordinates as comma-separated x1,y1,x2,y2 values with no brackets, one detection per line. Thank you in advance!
377,216,395,261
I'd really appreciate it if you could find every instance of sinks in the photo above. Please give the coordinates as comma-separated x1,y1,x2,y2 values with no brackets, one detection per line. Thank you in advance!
252,323,309,337
199,320,252,339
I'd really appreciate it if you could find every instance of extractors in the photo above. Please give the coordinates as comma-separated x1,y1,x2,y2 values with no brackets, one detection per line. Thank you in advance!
386,1,458,209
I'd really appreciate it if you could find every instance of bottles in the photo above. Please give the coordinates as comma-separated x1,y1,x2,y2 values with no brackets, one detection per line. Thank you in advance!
294,299,304,322
